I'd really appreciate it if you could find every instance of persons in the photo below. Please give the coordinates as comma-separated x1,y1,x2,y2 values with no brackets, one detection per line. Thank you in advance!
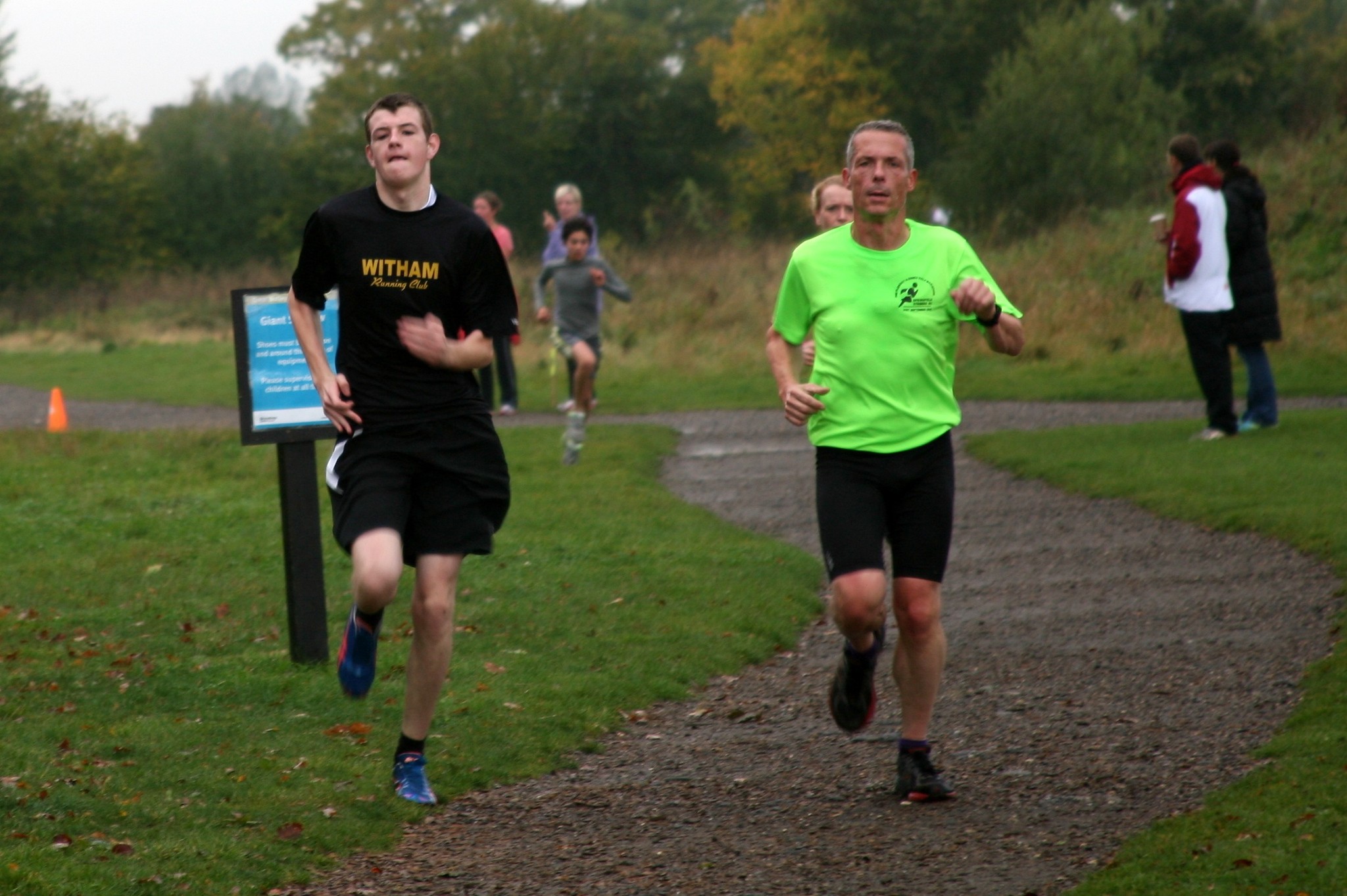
535,184,600,415
800,174,858,366
765,117,1026,807
287,93,515,806
1205,141,1283,433
1155,133,1238,441
459,191,521,419
535,219,630,468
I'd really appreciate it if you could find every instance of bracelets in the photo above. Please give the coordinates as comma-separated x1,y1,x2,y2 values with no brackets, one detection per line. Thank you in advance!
977,304,1002,329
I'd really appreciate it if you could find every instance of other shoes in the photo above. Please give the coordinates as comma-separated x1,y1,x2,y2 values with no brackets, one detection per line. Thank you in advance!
1238,415,1259,430
1202,427,1223,440
564,410,588,450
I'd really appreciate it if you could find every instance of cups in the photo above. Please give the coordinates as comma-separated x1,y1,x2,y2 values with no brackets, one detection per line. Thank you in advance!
1150,214,1167,241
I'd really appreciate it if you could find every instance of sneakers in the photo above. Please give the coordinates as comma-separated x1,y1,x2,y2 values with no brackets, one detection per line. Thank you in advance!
828,624,885,735
334,609,385,699
392,758,437,803
894,739,956,803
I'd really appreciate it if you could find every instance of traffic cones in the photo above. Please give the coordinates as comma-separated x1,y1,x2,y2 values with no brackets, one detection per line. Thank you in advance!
42,385,71,433
